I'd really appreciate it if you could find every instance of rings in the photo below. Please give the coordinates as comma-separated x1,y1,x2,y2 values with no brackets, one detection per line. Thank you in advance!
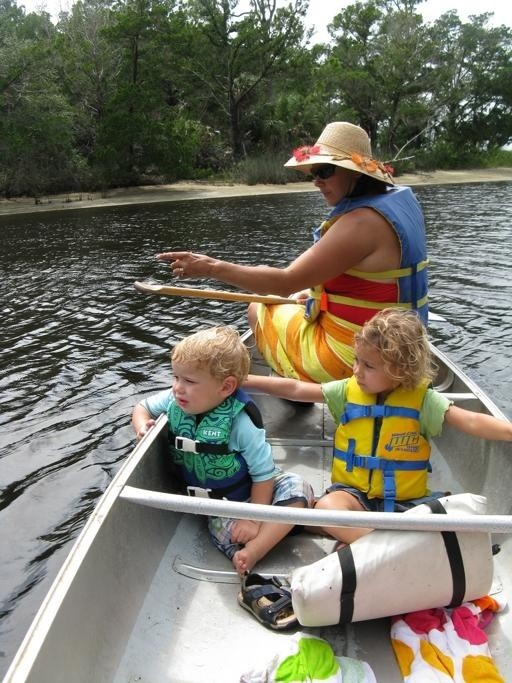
179,266,184,273
180,273,184,276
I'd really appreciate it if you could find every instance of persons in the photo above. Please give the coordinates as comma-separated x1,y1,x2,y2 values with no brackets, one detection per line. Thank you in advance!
130,328,316,579
239,307,511,554
157,121,427,406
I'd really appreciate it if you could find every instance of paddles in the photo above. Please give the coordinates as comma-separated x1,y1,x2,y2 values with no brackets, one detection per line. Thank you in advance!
134,281,446,323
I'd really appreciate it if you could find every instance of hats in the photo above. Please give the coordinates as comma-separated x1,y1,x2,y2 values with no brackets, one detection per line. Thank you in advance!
284,120,399,188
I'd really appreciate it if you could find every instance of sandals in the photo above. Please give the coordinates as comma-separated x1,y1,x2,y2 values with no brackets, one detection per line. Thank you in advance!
237,573,304,631
